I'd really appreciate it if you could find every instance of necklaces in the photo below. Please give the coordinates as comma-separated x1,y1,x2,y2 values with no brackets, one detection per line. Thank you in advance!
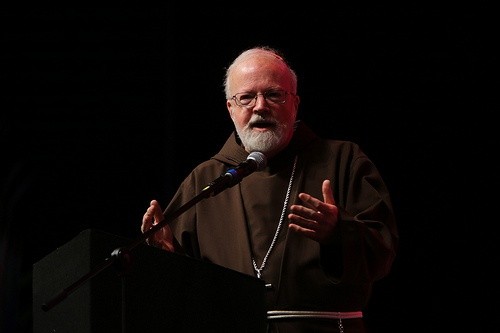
251,156,298,280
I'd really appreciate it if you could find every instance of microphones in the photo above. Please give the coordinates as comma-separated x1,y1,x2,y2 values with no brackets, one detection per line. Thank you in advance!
203,151,268,197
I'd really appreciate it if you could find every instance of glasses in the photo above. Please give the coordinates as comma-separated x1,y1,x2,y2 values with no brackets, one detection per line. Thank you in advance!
230,90,293,108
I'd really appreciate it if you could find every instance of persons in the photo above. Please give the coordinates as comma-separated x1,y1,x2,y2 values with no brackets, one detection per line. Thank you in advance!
140,48,394,333
